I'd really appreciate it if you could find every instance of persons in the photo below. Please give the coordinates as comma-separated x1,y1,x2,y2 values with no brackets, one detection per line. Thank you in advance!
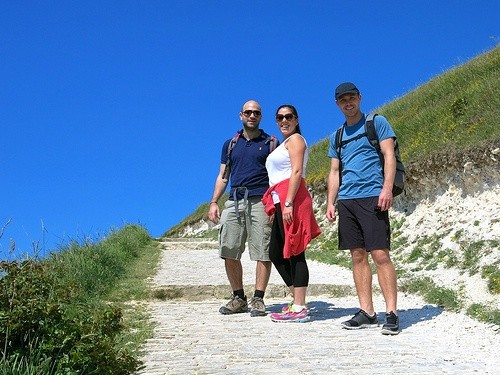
265,105,311,323
209,99,280,317
325,83,400,335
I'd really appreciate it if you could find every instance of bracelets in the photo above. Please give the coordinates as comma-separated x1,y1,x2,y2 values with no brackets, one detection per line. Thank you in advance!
210,201,217,205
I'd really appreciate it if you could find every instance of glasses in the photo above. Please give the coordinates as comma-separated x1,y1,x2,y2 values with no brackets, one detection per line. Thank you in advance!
277,113,294,121
243,110,261,117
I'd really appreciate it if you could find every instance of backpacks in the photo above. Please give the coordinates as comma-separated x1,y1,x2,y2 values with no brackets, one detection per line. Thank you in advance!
334,113,407,197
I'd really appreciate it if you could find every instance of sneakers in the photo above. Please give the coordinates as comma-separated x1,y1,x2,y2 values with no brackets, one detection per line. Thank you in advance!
247,296,266,317
281,302,312,315
270,302,310,323
340,308,379,329
381,310,401,334
218,293,248,315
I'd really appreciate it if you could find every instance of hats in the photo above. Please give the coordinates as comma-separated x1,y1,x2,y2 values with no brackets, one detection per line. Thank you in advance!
335,82,359,99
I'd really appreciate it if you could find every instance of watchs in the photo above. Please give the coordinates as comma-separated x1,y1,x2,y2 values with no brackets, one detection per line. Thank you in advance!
285,201,293,207
